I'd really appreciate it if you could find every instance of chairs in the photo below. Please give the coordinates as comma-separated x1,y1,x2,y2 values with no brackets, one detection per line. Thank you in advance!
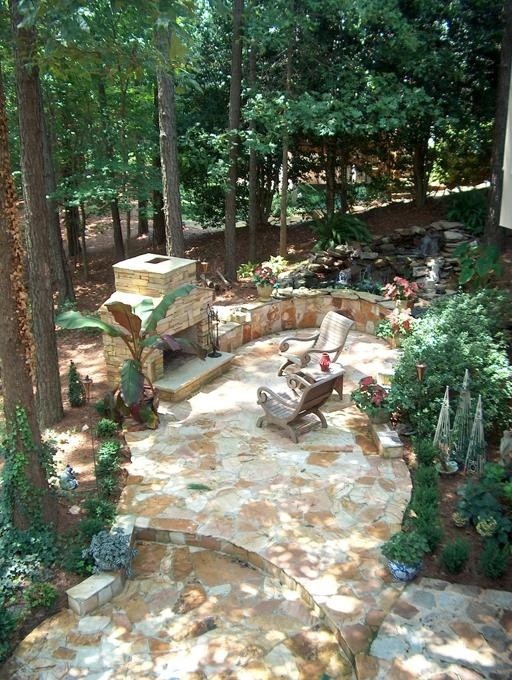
253,309,358,446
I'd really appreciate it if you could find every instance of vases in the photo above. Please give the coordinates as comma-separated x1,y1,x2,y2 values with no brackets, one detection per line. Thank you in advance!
366,404,392,425
257,285,272,297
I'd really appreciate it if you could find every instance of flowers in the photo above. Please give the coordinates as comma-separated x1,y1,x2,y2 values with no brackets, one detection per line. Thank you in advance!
235,253,289,286
376,309,416,339
381,275,417,302
348,377,397,414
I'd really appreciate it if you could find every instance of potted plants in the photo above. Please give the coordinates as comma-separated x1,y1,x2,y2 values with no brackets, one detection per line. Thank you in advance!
379,531,432,582
79,525,139,579
51,284,209,431
